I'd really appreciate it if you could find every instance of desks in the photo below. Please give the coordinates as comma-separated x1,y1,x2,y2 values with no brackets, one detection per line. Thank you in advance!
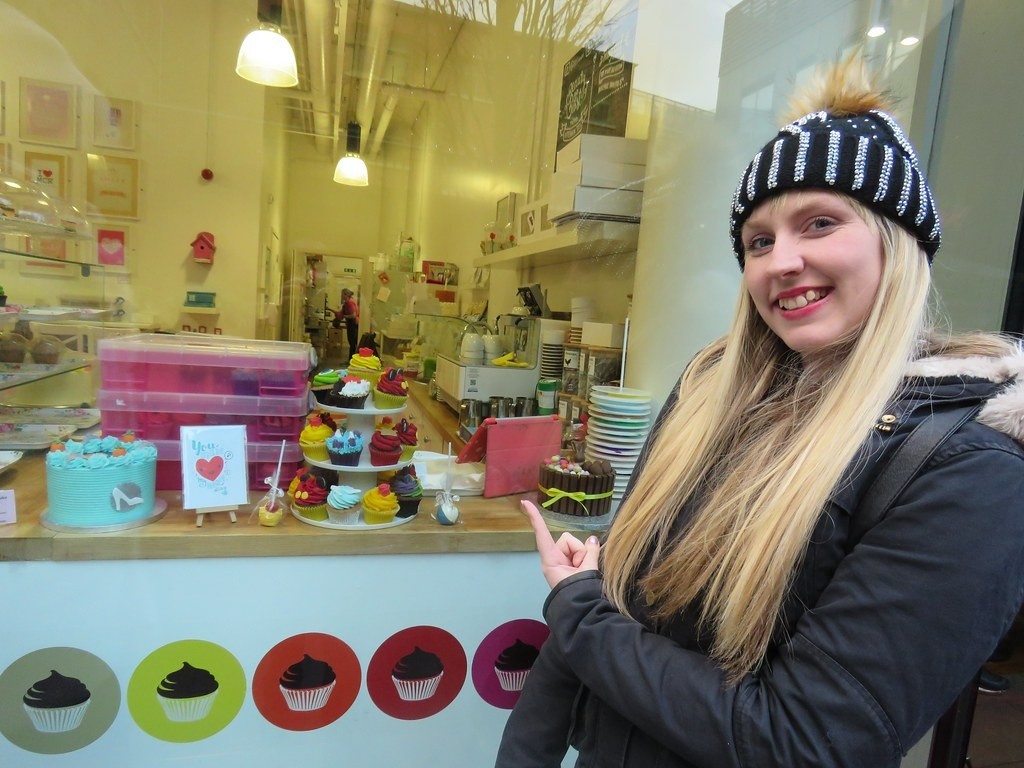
9,316,161,334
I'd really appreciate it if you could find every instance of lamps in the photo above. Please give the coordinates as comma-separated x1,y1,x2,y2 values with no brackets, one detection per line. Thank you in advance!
333,121,369,186
236,0,299,88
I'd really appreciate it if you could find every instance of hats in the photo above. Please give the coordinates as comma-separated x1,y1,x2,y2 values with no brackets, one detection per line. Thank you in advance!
729,46,942,271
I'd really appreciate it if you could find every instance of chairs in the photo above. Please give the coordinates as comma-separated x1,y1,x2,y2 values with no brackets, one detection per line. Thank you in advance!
31,322,85,353
86,326,140,356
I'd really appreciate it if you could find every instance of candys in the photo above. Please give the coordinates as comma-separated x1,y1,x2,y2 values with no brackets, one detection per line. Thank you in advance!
436,441,459,525
259,439,285,526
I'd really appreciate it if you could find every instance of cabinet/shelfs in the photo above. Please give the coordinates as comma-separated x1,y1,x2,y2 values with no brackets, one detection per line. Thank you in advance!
0,248,106,392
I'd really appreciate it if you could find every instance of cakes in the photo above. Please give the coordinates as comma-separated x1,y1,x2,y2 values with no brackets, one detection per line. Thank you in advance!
45,428,158,529
537,440,617,517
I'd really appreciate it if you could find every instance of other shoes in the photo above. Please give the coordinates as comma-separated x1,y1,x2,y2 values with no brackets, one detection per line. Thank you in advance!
340,361,349,366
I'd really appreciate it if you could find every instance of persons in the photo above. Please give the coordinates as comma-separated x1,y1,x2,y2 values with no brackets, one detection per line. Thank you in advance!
494,110,1024,768
327,288,359,365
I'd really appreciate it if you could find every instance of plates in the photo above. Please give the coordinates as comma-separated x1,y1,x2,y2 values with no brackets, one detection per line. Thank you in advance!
583,386,651,500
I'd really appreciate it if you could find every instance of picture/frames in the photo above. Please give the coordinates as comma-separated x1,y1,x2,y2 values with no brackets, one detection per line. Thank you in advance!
82,150,141,222
89,219,134,276
17,75,79,150
91,92,136,151
495,192,516,222
17,145,68,202
18,201,76,277
0,141,9,177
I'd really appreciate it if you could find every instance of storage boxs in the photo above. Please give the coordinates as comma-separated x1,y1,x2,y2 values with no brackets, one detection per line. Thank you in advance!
328,328,342,343
149,438,305,491
546,185,643,221
325,341,342,359
98,382,316,446
556,133,648,171
54,292,127,322
550,159,646,192
95,331,318,399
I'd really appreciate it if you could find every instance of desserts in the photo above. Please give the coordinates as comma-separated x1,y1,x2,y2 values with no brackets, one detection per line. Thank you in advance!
0,319,65,365
310,347,410,410
157,661,218,723
278,654,337,711
22,669,91,733
299,409,419,468
392,645,443,701
287,460,424,525
493,639,540,691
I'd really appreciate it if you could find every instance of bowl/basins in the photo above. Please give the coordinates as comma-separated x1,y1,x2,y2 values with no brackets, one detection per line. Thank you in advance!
461,334,504,366
